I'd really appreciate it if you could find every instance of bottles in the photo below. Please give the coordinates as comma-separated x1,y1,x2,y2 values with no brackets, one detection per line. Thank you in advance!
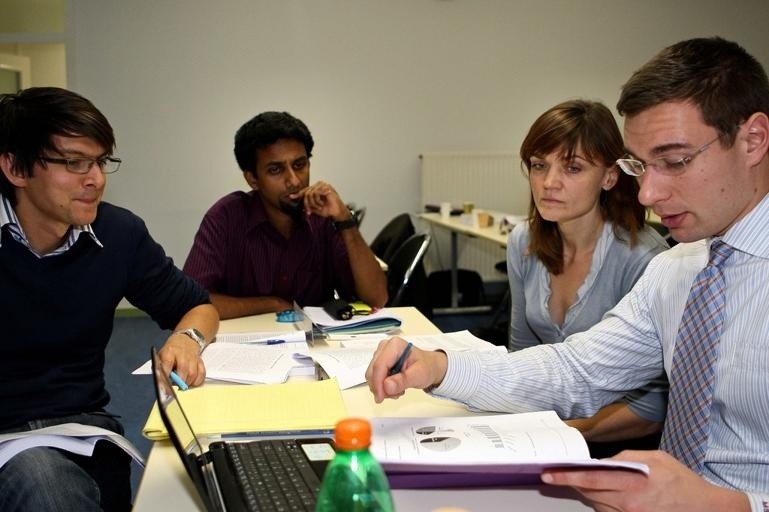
314,417,394,512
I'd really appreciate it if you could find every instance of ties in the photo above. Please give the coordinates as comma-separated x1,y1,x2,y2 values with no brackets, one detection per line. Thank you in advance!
658,241,734,475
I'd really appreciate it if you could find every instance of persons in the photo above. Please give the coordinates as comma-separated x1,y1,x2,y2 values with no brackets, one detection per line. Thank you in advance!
0,83,221,512
183,111,389,321
364,36,769,511
503,98,672,441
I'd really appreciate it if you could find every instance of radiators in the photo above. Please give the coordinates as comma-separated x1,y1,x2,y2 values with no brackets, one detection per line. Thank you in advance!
417,149,534,286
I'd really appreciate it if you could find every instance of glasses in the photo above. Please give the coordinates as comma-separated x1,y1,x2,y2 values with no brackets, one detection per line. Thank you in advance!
38,153,120,174
615,132,725,175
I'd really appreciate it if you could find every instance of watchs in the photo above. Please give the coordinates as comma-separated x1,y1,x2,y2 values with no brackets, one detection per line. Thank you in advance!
171,328,206,355
332,208,359,234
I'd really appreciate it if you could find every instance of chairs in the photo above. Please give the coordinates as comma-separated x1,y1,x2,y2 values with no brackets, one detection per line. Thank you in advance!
369,212,416,263
382,232,431,307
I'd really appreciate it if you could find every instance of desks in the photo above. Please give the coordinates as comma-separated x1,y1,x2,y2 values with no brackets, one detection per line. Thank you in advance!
417,202,528,314
131,307,597,510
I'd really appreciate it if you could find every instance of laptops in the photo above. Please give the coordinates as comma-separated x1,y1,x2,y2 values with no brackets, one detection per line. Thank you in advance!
149,346,386,512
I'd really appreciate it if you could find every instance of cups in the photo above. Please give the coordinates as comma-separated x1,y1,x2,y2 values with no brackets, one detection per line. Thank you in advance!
440,202,451,218
478,212,490,228
463,202,475,213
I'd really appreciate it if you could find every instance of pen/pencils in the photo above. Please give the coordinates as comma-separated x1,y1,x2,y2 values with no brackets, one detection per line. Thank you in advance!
170,371,189,391
390,343,414,376
243,340,284,345
